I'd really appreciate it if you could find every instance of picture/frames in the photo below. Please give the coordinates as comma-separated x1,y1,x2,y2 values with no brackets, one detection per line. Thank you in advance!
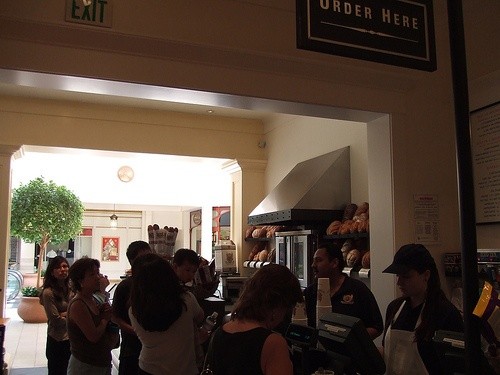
101,236,120,261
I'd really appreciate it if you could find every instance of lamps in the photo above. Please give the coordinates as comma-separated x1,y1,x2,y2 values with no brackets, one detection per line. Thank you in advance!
110,205,118,227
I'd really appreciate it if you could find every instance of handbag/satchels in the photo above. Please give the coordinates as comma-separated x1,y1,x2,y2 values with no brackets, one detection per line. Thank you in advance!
91,295,120,348
198,329,221,375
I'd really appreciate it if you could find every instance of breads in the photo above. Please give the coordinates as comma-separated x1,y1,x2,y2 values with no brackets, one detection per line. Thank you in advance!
245,225,287,239
331,238,370,269
326,202,369,236
247,241,276,262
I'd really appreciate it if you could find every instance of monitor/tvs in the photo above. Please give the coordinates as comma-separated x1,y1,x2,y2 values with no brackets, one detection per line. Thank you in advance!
432,330,466,375
317,312,386,375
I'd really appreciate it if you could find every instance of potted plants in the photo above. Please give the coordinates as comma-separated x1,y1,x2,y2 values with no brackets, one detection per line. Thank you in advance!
18,287,49,323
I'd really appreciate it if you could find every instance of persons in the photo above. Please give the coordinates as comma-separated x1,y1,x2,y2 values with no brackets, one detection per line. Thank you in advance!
379,242,467,375
127,251,206,375
111,241,154,375
199,263,305,375
302,243,385,341
66,257,121,375
39,254,76,374
169,248,201,284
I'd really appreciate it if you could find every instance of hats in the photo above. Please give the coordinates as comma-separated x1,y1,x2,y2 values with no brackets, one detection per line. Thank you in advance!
382,243,434,274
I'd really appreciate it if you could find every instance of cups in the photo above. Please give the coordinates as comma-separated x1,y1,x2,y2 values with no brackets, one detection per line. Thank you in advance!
316,278,333,327
292,296,308,327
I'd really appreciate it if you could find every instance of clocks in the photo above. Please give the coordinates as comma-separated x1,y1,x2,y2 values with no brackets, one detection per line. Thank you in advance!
118,166,134,182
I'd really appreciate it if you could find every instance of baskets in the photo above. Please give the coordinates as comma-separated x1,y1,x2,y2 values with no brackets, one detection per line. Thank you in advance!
192,271,222,299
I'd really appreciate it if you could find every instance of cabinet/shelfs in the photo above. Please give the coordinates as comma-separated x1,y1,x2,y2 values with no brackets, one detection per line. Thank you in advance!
242,228,370,292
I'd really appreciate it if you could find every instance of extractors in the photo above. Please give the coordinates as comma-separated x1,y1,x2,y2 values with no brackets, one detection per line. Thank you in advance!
247,146,350,226
